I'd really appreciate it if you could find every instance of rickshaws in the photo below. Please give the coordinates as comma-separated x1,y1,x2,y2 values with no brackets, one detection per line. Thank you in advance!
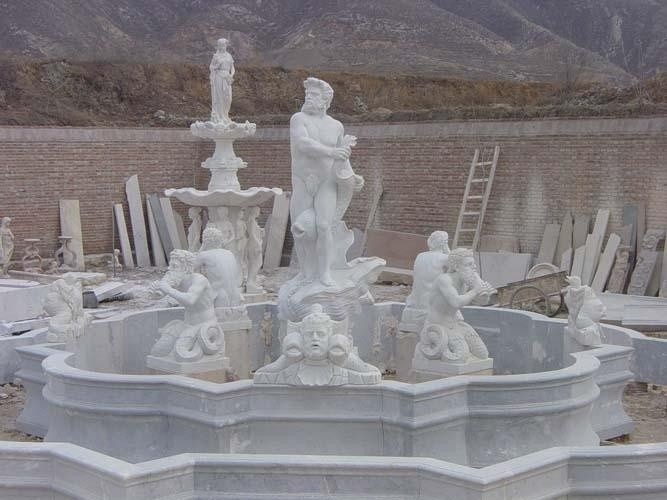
482,269,571,319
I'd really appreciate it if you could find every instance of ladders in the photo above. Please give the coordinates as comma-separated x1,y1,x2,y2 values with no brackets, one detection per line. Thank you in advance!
452,145,500,250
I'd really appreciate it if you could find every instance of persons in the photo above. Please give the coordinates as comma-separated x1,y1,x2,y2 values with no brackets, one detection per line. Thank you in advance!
252,309,382,387
564,275,602,347
400,231,453,331
186,206,266,293
423,247,495,362
289,77,365,287
0,216,16,279
196,228,248,322
144,247,227,359
208,37,236,124
